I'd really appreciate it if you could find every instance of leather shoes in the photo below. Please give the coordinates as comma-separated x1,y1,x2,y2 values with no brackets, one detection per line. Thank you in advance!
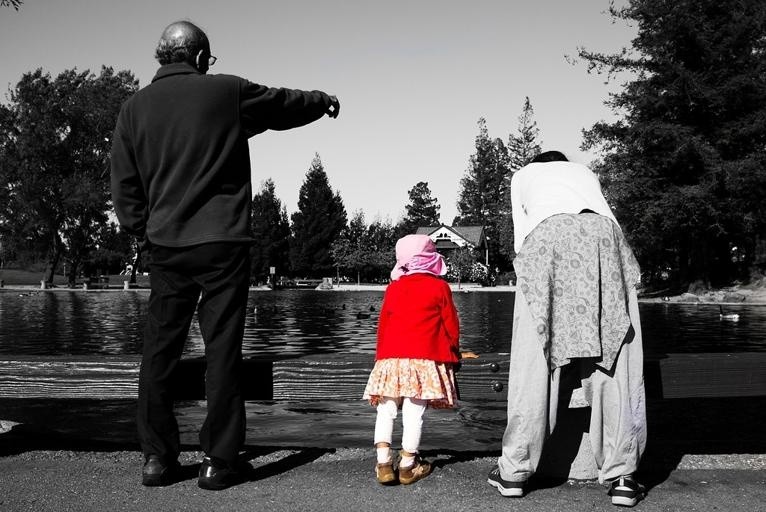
197,454,253,490
142,454,180,487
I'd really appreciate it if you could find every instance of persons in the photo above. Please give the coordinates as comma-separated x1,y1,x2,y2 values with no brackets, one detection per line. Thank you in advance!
360,234,479,485
110,21,340,490
487,150,647,506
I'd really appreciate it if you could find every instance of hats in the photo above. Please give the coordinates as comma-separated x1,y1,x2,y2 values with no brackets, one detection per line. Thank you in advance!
390,233,448,281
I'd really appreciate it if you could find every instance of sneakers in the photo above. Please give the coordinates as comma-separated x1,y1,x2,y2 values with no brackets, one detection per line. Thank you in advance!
487,468,525,497
374,461,432,485
611,477,647,507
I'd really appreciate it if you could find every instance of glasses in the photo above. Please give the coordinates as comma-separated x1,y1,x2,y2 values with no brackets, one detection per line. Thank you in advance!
208,54,217,66
